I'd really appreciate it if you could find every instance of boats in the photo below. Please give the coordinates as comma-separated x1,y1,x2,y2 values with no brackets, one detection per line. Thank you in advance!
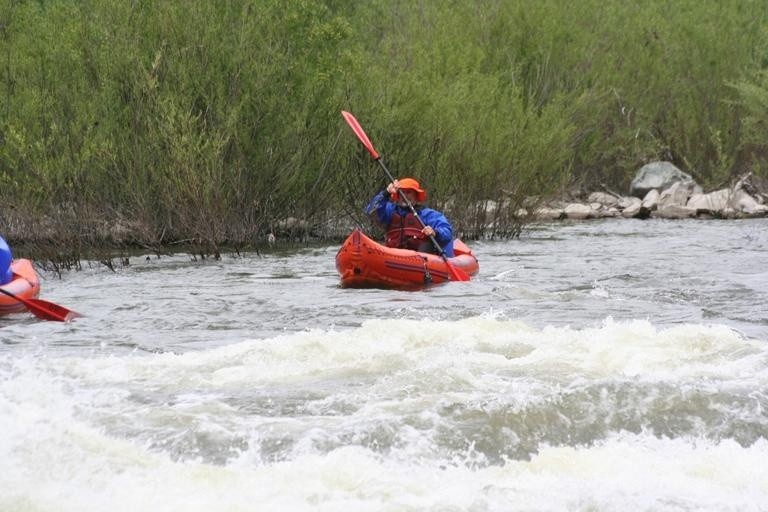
332,228,480,293
0,252,42,314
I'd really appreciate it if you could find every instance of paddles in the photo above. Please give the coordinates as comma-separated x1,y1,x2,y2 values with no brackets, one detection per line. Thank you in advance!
0,287,86,324
340,110,471,282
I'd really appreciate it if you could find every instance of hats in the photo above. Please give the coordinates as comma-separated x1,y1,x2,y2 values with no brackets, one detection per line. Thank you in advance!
390,178,425,202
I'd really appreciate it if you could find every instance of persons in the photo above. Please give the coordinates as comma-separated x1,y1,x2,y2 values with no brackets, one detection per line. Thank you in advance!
364,176,452,261
